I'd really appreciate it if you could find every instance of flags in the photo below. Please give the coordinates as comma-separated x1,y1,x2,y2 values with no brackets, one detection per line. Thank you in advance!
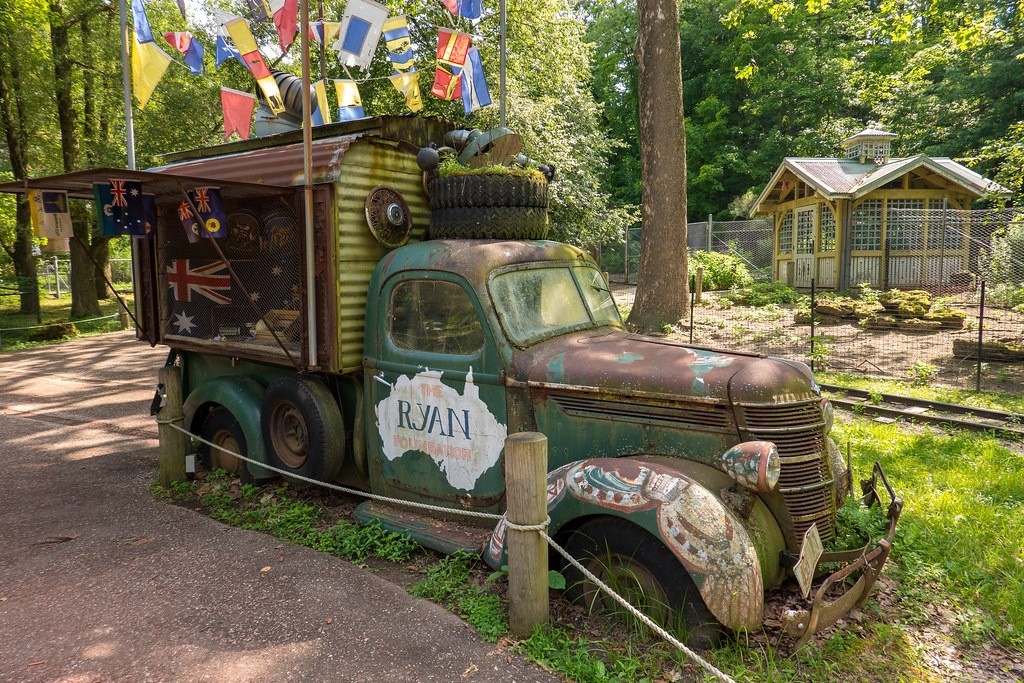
451,49,491,113
310,0,390,68
382,18,414,70
29,190,74,238
443,0,483,19
388,72,423,111
431,66,462,99
163,0,298,139
92,179,158,237
165,260,243,335
334,80,364,121
437,29,470,66
177,187,225,244
310,80,332,127
126,0,172,109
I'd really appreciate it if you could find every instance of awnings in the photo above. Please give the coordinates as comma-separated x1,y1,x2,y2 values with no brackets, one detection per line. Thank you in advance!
0,167,301,369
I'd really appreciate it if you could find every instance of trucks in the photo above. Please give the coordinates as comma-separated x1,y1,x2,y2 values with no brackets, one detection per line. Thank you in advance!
1,66,902,658
